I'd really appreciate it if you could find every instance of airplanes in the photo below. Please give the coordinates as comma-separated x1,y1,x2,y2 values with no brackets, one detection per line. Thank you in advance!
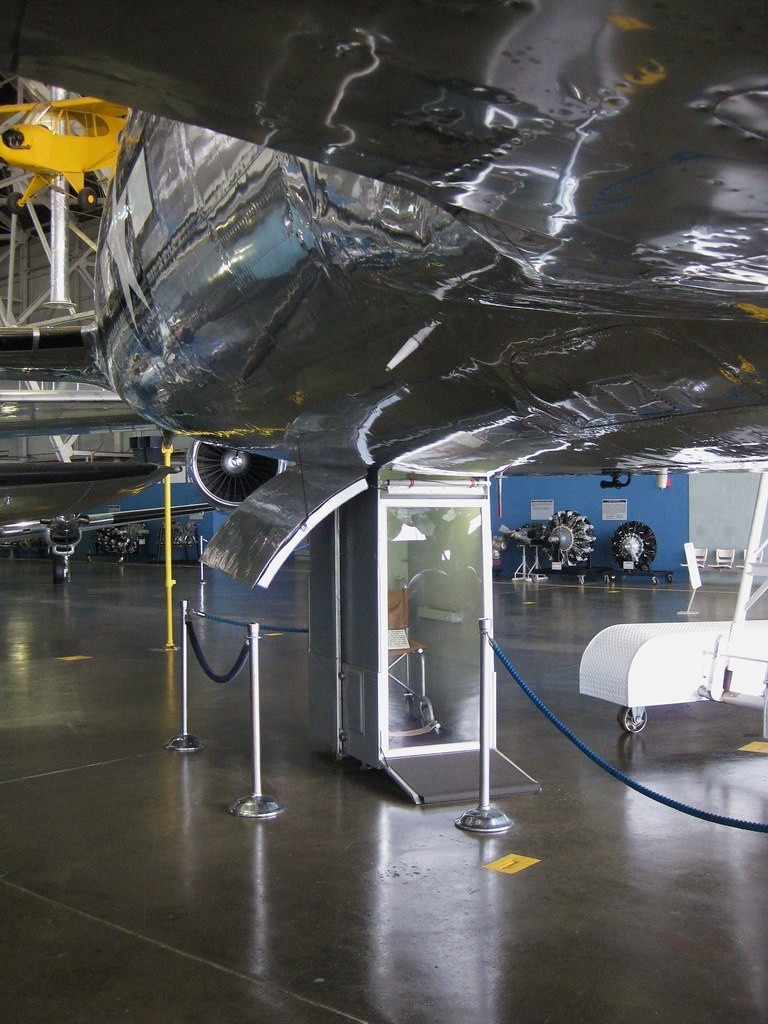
0,0,768,582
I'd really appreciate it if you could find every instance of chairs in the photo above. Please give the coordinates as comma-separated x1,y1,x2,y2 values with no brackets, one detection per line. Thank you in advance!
708,549,735,569
680,547,708,568
388,576,441,734
736,549,764,570
